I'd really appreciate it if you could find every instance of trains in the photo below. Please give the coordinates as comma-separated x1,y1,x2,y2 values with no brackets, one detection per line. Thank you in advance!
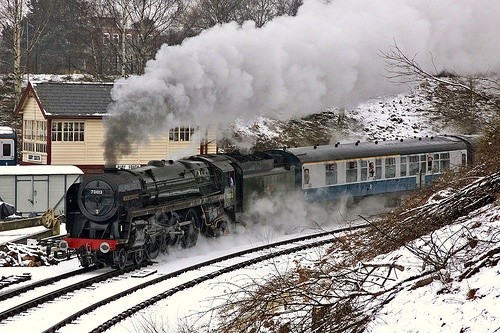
55,133,489,276
